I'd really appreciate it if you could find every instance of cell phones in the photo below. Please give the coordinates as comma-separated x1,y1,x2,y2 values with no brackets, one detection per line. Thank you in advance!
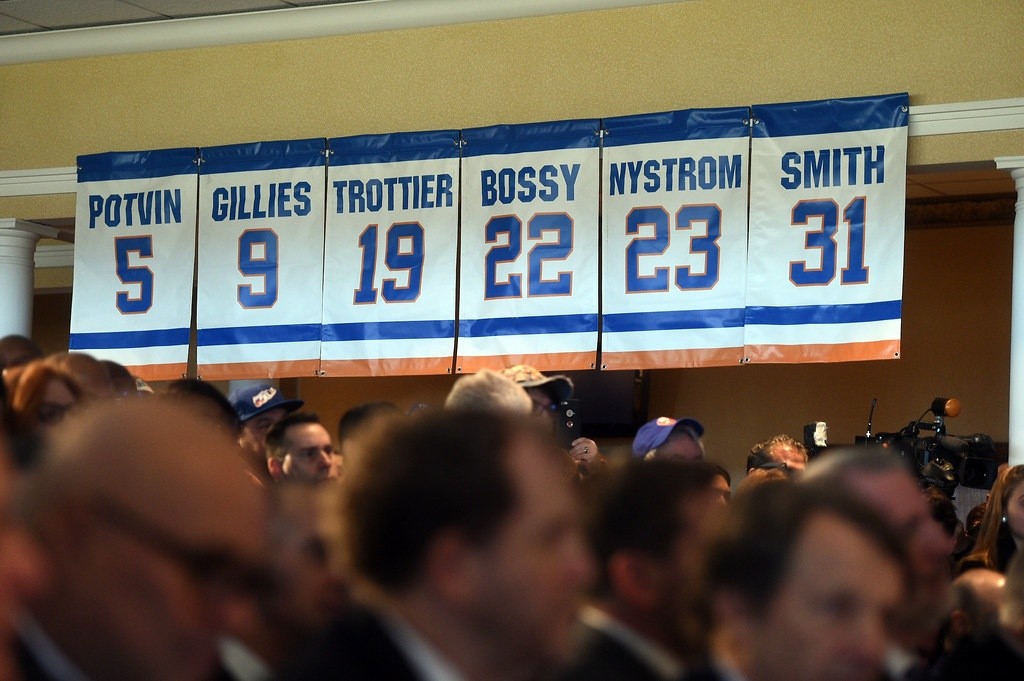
556,399,581,448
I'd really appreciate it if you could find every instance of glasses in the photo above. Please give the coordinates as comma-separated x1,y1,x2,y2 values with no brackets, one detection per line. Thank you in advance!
80,489,278,614
34,394,80,426
531,399,568,418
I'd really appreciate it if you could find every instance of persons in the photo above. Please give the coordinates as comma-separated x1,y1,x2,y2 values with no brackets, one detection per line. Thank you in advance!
0,335,1024,681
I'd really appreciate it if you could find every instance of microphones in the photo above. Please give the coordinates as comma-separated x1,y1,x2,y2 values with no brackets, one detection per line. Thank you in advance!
938,435,970,453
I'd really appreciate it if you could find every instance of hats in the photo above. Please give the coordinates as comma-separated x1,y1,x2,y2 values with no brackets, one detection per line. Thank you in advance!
502,366,576,401
631,416,705,461
227,380,306,421
